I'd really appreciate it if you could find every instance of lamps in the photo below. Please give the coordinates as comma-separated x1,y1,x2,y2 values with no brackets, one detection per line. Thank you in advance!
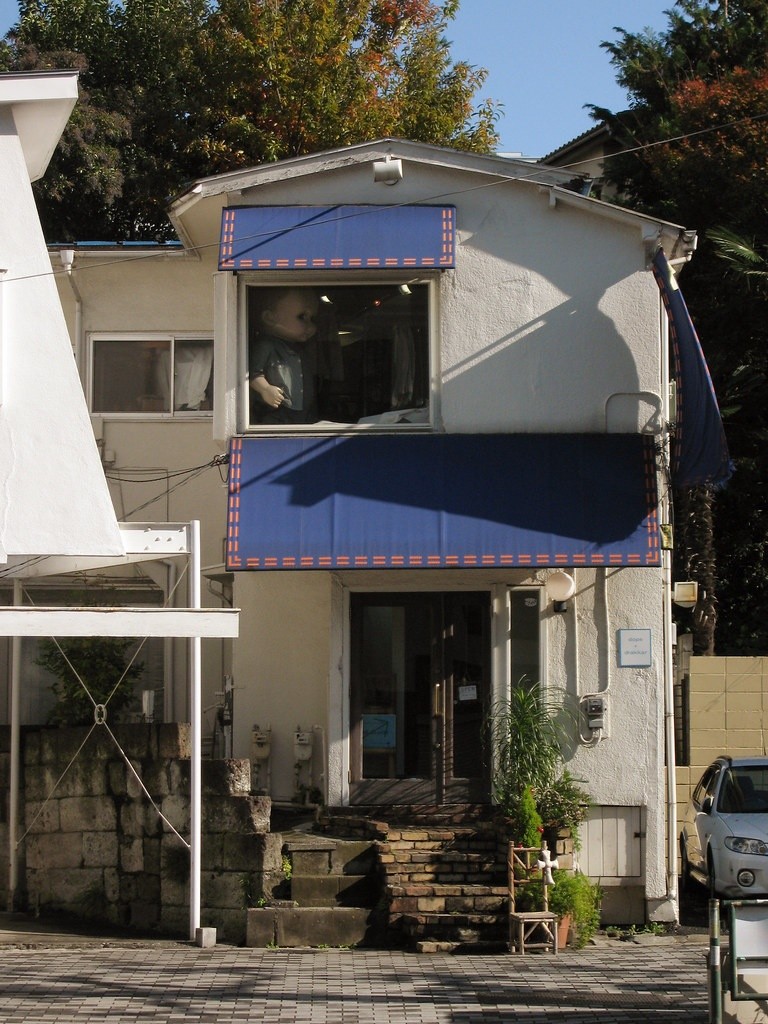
547,571,575,613
373,159,403,181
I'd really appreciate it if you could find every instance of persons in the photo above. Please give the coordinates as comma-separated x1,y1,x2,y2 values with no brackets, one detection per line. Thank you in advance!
248,287,319,423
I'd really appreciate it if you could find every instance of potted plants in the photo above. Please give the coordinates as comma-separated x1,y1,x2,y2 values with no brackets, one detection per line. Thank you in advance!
506,770,602,949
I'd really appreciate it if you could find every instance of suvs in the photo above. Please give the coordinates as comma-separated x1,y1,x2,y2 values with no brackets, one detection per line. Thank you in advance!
679,754,768,917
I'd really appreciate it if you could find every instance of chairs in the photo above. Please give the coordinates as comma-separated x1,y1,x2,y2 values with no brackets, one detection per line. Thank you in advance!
508,841,559,956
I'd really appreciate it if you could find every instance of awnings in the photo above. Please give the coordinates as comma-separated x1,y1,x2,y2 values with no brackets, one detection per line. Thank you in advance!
227,426,662,571
216,201,458,272
